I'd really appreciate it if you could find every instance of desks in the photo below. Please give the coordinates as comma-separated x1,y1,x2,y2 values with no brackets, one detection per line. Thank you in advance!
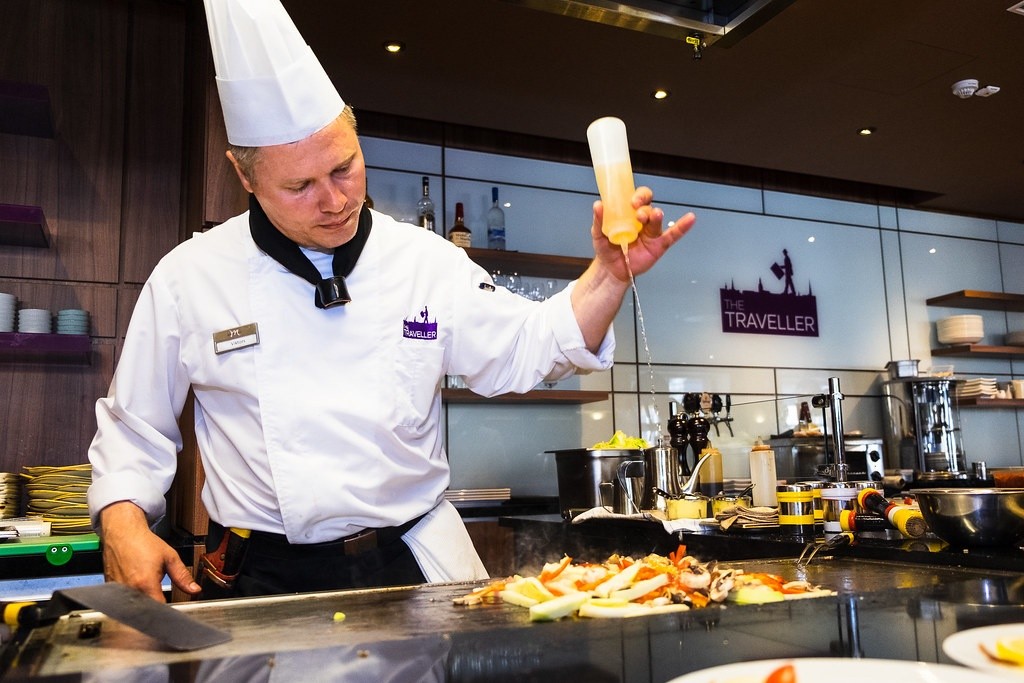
499,510,1024,590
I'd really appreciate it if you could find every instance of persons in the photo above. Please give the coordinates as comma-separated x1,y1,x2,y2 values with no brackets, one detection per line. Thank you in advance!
88,89,695,603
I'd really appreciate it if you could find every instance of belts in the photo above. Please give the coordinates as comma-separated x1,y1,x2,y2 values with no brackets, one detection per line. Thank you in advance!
253,513,427,558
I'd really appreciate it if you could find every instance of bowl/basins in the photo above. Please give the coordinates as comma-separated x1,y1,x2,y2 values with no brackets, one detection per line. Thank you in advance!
985,466,1024,488
664,495,708,520
909,486,1024,552
598,474,698,516
709,494,752,521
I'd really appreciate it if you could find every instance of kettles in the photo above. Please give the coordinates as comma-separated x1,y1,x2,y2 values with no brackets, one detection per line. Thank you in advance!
879,375,968,474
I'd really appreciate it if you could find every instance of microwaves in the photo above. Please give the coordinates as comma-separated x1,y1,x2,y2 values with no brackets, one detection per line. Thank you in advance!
769,435,885,484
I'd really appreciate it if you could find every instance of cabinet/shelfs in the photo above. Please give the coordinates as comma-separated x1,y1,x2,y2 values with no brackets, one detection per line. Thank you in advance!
0,201,94,372
433,241,609,406
0,530,195,624
924,288,1024,409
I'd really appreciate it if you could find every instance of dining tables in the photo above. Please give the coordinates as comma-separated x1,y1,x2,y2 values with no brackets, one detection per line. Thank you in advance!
0,586,1024,683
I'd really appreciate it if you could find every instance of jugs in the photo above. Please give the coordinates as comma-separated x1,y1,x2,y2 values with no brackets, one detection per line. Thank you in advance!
616,436,713,516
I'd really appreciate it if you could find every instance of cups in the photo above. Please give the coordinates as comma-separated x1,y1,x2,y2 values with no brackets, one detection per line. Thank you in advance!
440,373,468,389
776,481,885,537
491,269,558,303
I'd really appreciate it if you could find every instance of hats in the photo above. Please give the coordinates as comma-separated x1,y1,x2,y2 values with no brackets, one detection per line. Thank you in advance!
203,0,345,147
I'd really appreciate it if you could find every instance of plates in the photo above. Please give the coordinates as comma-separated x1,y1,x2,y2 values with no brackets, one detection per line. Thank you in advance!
940,622,1024,683
936,314,985,346
954,376,998,400
442,486,511,508
56,308,90,335
0,472,21,520
25,462,94,535
1003,330,1024,346
0,292,17,332
665,656,1024,683
17,308,52,334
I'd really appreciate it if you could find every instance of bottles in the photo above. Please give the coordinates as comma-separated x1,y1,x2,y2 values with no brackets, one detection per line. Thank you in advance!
486,187,506,250
749,436,779,509
1005,384,1013,399
586,115,643,257
698,440,724,499
363,176,375,210
882,475,906,489
447,202,472,249
417,175,436,233
